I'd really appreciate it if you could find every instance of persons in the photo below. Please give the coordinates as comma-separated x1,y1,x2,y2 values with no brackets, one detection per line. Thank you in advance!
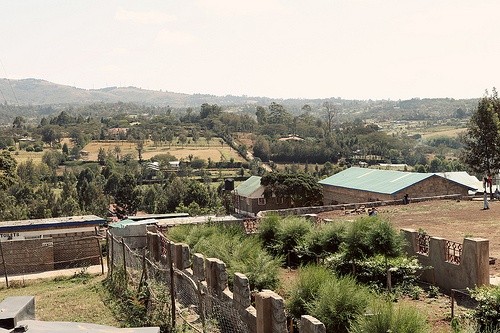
494,188,500,201
403,193,410,205
368,206,378,218
480,190,490,210
